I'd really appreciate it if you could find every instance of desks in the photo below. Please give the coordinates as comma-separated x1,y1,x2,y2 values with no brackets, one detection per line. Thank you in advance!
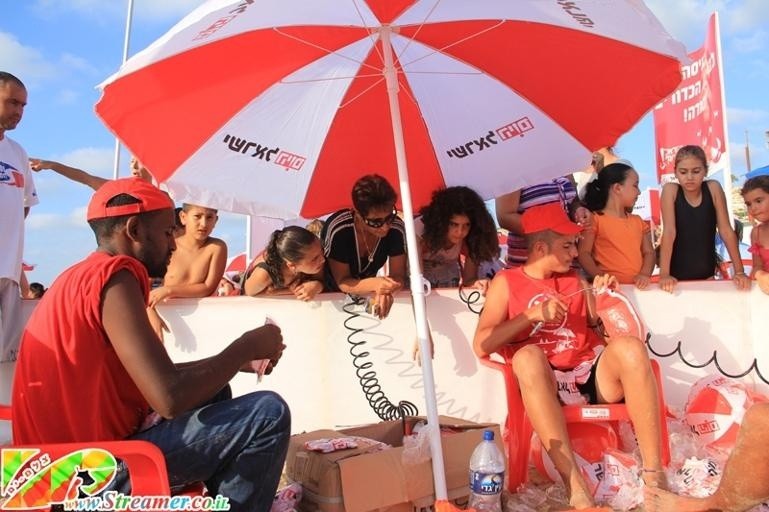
0,276,769,450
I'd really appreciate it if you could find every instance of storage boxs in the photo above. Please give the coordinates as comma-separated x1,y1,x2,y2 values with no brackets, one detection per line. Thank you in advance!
284,413,510,510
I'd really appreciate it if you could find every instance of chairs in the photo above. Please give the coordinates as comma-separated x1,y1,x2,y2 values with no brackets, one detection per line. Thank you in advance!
0,399,207,510
474,334,673,493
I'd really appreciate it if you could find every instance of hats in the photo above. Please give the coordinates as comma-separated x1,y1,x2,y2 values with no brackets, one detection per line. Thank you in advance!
522,202,587,235
88,178,175,220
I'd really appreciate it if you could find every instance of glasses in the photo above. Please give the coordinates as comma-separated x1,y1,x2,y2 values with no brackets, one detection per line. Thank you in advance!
363,204,397,227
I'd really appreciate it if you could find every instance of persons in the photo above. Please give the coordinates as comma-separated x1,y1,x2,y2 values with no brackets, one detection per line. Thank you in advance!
471,201,671,512
28,281,48,299
26,151,156,190
144,141,769,366
1,72,38,408
640,402,768,512
8,178,291,511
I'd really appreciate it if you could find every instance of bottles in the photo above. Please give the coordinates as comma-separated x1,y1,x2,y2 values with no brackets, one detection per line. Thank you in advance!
465,429,505,512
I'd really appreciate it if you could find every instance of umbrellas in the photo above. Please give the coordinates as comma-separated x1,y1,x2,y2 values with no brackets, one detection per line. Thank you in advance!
93,0,691,500
